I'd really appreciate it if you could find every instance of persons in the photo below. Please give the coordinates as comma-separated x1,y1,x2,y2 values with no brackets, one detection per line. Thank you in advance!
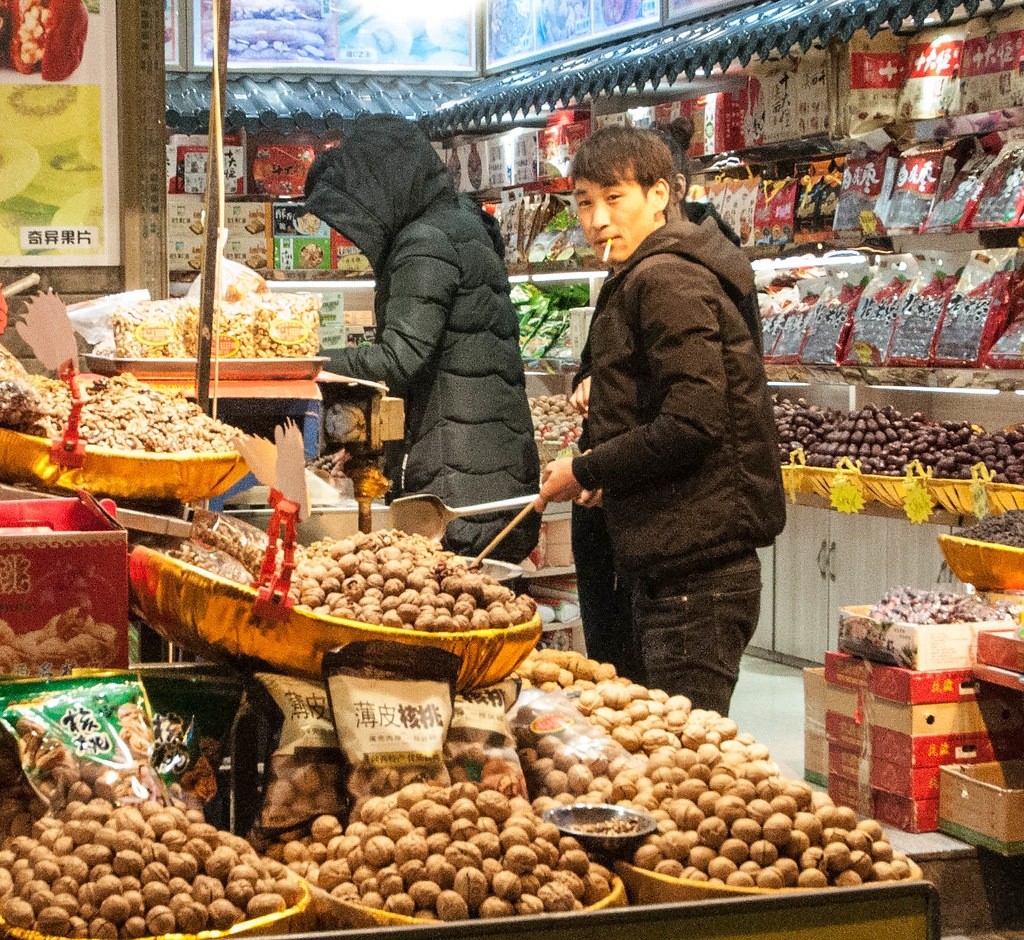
533,118,788,720
794,170,842,232
317,114,542,566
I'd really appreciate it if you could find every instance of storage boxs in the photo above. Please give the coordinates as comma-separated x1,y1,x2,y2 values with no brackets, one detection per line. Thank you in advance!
272,201,331,237
977,628,1024,674
463,139,492,193
827,741,942,834
487,127,543,188
546,110,591,128
824,651,997,768
165,126,274,274
886,517,965,593
274,234,330,269
802,667,829,789
0,486,129,680
446,146,466,194
836,604,1016,671
937,759,1024,858
537,118,591,180
654,92,735,156
331,228,373,269
295,291,377,351
250,144,315,194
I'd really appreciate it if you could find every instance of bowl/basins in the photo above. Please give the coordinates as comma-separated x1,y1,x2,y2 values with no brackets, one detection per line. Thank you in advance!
544,803,656,854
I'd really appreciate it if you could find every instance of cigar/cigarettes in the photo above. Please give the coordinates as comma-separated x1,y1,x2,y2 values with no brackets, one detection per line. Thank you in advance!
603,239,611,262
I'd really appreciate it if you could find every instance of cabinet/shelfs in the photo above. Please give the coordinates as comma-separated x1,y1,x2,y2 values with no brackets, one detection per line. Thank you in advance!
594,112,631,130
773,502,906,668
479,105,1024,433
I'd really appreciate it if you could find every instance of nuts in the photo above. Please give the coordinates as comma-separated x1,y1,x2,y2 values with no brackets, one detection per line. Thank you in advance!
0,645,910,940
0,274,326,456
525,393,586,442
137,508,539,635
